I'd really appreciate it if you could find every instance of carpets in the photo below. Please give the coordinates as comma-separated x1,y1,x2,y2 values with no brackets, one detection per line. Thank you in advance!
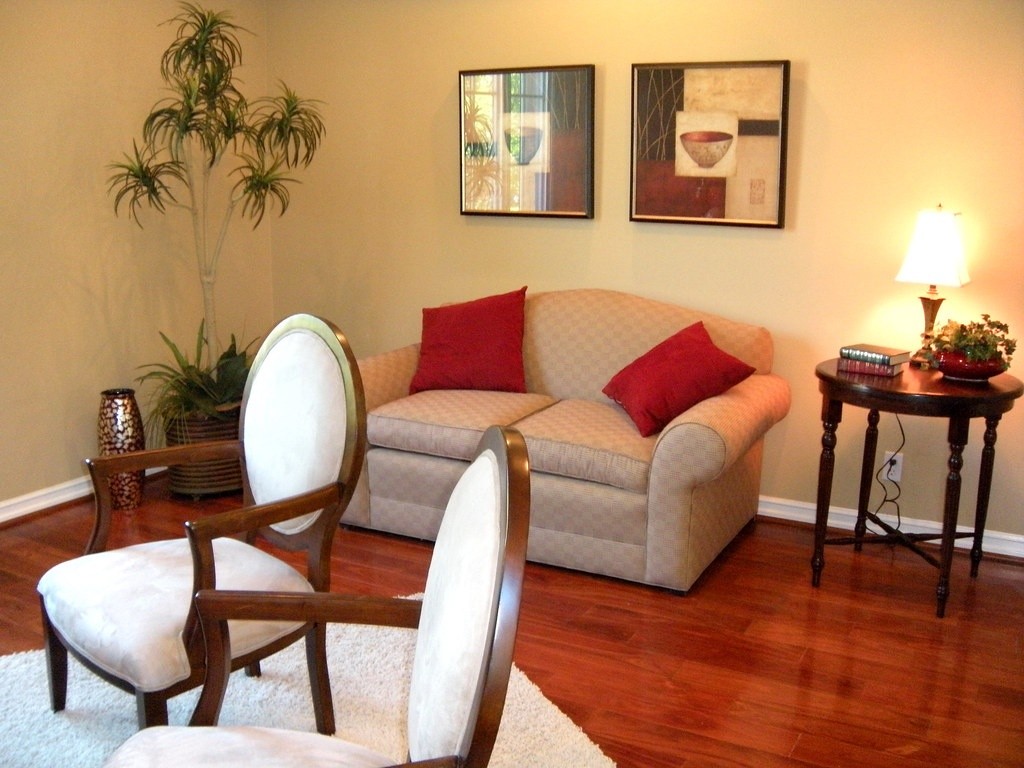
1,621,619,768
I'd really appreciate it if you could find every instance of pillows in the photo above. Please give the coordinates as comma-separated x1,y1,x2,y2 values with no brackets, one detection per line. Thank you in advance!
409,285,529,396
602,321,757,438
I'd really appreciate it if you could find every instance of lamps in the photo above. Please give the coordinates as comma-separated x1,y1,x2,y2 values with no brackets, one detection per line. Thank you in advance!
896,202,972,365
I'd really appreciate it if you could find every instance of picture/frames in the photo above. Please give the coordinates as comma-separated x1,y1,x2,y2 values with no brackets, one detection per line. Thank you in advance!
459,64,595,219
629,59,790,228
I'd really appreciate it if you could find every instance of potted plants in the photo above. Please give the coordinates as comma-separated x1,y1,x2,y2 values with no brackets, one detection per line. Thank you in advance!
921,313,1018,382
105,0,330,499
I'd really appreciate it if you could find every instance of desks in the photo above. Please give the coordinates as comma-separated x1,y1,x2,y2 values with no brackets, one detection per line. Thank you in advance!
811,354,1024,617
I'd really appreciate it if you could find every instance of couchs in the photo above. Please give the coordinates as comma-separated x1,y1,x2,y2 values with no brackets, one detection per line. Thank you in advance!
337,288,792,598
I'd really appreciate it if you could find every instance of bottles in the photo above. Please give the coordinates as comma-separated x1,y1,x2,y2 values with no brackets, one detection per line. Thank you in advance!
98,389,145,510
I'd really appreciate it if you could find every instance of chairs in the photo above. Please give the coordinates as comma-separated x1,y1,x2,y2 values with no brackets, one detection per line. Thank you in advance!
37,311,367,733
104,426,531,768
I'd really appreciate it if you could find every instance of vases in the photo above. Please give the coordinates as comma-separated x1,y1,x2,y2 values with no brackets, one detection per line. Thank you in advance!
98,387,146,509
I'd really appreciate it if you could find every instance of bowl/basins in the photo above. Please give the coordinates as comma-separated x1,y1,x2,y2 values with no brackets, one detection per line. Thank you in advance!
503,126,543,166
679,131,734,169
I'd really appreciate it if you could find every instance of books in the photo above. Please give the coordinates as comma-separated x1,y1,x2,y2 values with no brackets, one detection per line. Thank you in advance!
837,343,911,376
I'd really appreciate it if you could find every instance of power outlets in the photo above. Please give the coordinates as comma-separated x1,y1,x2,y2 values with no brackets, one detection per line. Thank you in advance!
883,451,904,481
